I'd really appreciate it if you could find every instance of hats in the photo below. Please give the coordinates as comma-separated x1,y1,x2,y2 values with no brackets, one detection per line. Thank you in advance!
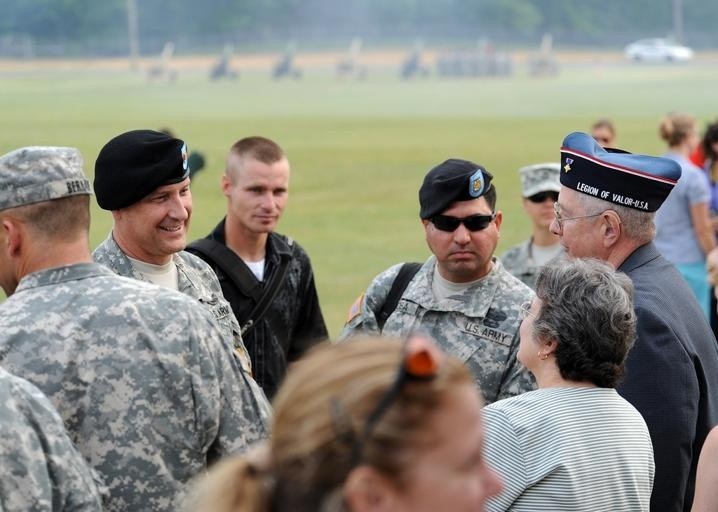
419,160,493,220
1,146,93,213
520,163,560,197
93,129,190,211
560,131,683,212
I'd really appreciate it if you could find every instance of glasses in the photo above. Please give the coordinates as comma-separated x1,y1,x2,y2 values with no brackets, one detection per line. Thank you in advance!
432,212,496,231
531,191,559,204
553,201,621,229
518,301,536,321
347,335,442,473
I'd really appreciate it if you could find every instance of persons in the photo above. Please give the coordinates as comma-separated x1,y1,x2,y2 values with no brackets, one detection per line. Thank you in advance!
175,335,504,511
501,163,564,294
697,119,717,177
0,369,110,512
335,159,540,410
0,144,276,512
653,113,717,264
184,135,328,405
476,256,655,511
594,122,617,149
688,247,718,511
89,130,275,435
549,133,718,511
